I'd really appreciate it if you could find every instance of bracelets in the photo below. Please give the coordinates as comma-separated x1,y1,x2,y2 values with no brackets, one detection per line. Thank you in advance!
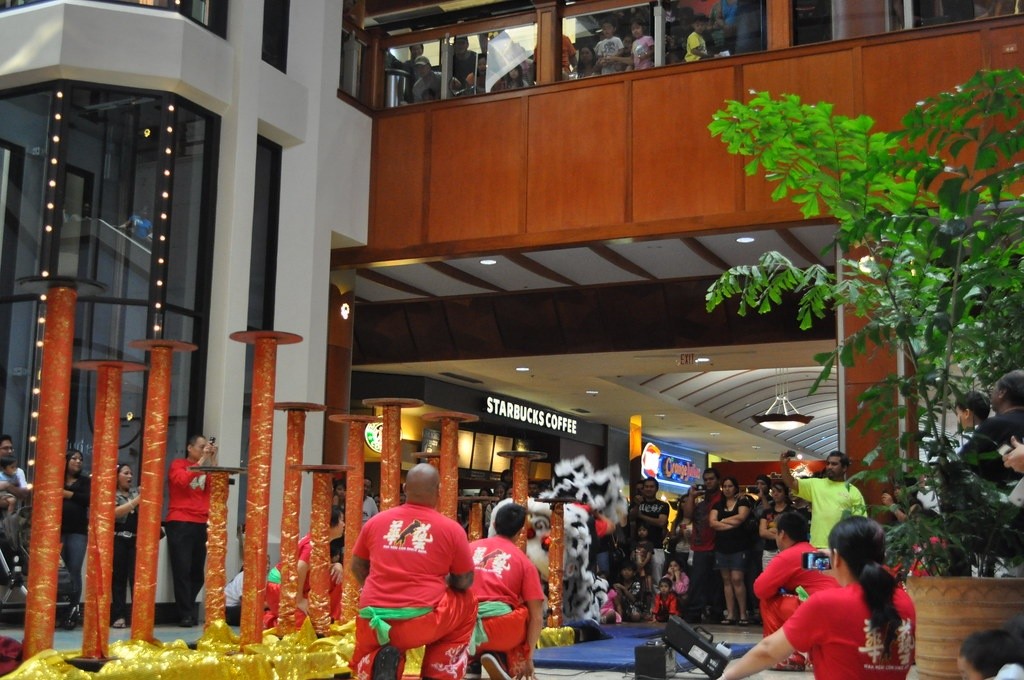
129,501,134,509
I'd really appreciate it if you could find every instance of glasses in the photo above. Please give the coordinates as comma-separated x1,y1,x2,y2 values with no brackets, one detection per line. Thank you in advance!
0,446,14,450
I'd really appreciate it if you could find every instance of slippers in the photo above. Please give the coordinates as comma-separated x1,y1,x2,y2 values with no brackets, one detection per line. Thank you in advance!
738,619,750,626
112,620,128,629
720,618,737,625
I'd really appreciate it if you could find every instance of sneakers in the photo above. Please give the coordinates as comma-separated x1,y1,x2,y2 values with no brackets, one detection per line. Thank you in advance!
370,646,403,680
480,652,512,680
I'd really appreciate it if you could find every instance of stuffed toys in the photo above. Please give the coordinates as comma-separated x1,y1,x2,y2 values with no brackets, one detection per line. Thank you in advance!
488,458,628,637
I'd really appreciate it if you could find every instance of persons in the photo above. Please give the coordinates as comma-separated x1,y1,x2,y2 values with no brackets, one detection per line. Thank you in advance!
110,464,140,628
225,556,270,626
572,0,763,78
780,450,868,548
596,468,814,624
458,469,549,534
893,0,1024,27
349,464,479,680
383,27,577,103
958,614,1024,680
812,558,829,569
0,434,28,516
883,371,1024,580
753,514,841,671
167,436,218,626
264,506,345,630
331,477,406,564
469,504,545,680
717,516,915,680
60,450,90,630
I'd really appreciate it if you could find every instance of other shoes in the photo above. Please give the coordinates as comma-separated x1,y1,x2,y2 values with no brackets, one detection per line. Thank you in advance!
179,614,199,628
766,658,806,672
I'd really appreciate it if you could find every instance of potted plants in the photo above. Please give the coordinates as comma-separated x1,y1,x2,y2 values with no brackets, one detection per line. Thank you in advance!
705,65,1024,680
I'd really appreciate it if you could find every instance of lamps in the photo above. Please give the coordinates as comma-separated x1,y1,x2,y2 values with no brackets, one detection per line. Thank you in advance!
751,367,814,432
